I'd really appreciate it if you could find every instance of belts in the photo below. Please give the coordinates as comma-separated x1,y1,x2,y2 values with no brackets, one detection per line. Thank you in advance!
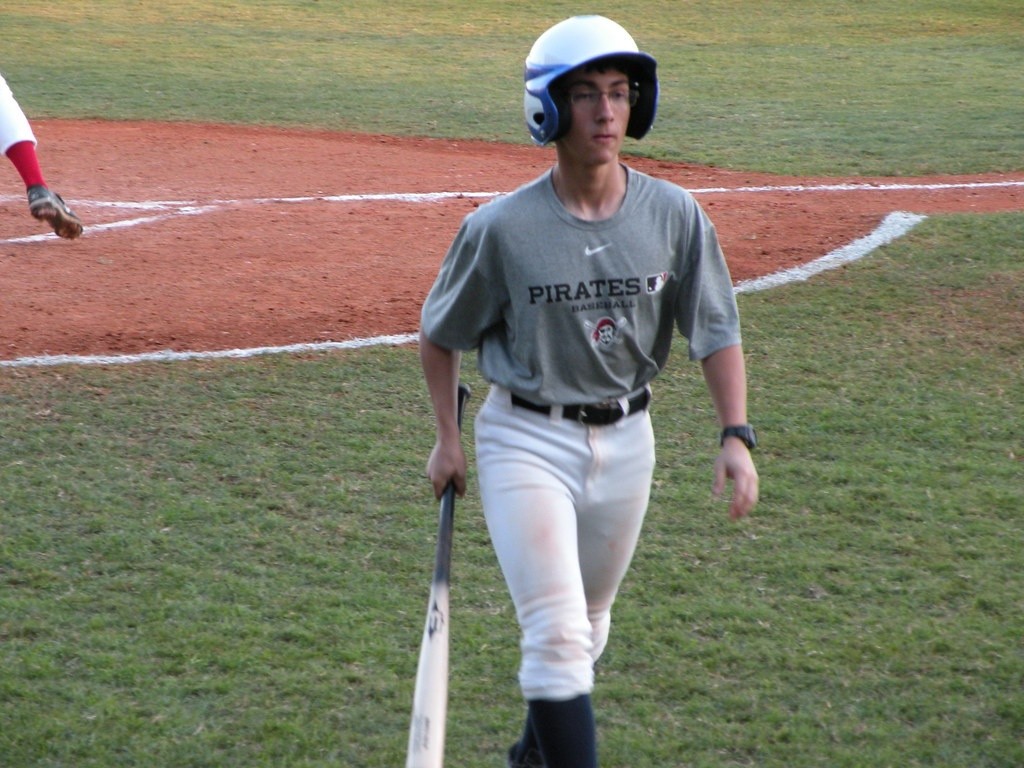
508,389,653,424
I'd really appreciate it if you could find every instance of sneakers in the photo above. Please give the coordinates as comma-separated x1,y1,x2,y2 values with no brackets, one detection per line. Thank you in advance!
26,185,84,239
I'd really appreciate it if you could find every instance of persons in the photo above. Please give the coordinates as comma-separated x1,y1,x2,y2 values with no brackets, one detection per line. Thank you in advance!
418,16,758,768
0,75,83,240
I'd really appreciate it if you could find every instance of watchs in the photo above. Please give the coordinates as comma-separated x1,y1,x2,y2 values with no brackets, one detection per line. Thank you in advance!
719,426,758,450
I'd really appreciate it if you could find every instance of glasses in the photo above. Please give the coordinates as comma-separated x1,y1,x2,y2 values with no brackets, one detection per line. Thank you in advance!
560,79,642,111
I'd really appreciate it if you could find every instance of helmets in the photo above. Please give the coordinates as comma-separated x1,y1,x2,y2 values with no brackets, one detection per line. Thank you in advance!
521,15,660,147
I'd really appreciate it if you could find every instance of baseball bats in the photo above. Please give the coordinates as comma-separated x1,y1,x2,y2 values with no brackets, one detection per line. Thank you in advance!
405,379,477,768
